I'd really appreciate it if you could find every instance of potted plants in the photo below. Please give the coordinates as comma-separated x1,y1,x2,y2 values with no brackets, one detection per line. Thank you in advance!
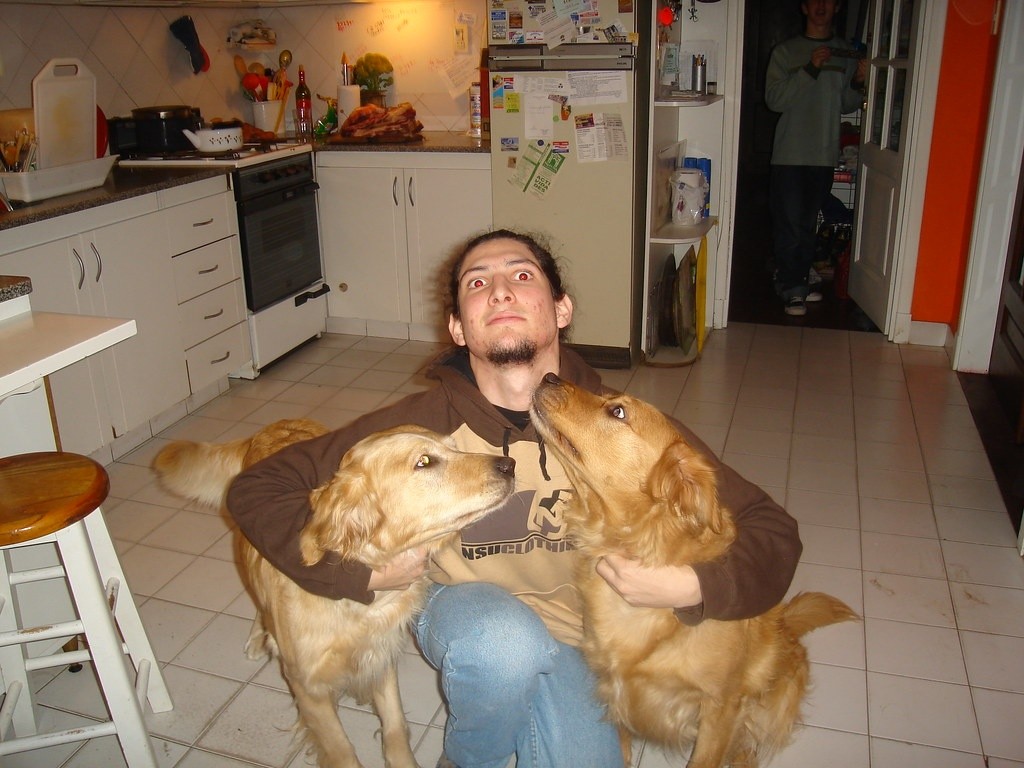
350,53,394,108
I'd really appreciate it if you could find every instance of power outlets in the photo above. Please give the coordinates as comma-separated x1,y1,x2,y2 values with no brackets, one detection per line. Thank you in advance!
452,23,469,53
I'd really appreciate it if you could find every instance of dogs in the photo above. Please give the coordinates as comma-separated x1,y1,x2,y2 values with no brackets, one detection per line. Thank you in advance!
152,415,518,768
528,373,863,768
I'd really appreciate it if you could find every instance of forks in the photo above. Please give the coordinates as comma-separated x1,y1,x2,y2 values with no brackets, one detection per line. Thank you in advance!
18,143,29,170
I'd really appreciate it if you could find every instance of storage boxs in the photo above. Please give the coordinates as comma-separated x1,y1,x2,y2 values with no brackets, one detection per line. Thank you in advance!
0,154,120,202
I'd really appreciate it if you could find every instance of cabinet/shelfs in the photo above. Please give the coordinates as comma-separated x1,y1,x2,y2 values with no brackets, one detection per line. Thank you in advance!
639,0,724,367
0,151,492,467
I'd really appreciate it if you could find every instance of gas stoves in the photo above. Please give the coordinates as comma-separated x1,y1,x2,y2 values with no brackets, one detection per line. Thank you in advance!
107,116,313,171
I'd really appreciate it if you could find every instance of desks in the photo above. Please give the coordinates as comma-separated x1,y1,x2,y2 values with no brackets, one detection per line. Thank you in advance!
0,311,137,397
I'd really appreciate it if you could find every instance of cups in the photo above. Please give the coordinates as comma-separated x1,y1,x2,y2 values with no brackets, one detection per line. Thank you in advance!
337,83,360,134
693,65,707,97
253,99,286,133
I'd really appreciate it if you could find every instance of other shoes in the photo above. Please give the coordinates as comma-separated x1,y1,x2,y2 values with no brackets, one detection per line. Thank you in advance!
806,289,823,302
784,295,807,316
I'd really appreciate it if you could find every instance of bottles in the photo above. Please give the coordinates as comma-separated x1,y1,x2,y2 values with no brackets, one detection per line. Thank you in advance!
469,82,482,138
296,64,313,136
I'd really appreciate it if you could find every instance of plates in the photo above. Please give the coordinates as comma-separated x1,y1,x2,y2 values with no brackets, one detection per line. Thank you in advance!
96,105,108,158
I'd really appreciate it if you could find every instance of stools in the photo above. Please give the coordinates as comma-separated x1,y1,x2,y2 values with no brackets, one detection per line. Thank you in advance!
0,452,174,768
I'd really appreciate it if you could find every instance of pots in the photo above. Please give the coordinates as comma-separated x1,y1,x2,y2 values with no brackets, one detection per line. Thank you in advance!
132,104,191,153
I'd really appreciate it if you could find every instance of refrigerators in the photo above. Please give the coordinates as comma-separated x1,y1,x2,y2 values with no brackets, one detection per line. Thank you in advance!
489,0,652,371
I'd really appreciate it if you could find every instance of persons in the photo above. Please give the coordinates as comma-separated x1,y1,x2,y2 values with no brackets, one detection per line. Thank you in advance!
764,1,866,314
227,229,802,768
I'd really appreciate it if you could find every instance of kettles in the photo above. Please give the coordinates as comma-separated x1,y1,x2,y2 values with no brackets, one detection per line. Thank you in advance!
182,120,244,153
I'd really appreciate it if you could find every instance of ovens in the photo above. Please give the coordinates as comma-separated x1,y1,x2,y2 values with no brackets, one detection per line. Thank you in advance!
229,152,325,380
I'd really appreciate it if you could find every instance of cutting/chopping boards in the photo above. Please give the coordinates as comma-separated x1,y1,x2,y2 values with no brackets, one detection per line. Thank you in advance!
31,57,97,170
